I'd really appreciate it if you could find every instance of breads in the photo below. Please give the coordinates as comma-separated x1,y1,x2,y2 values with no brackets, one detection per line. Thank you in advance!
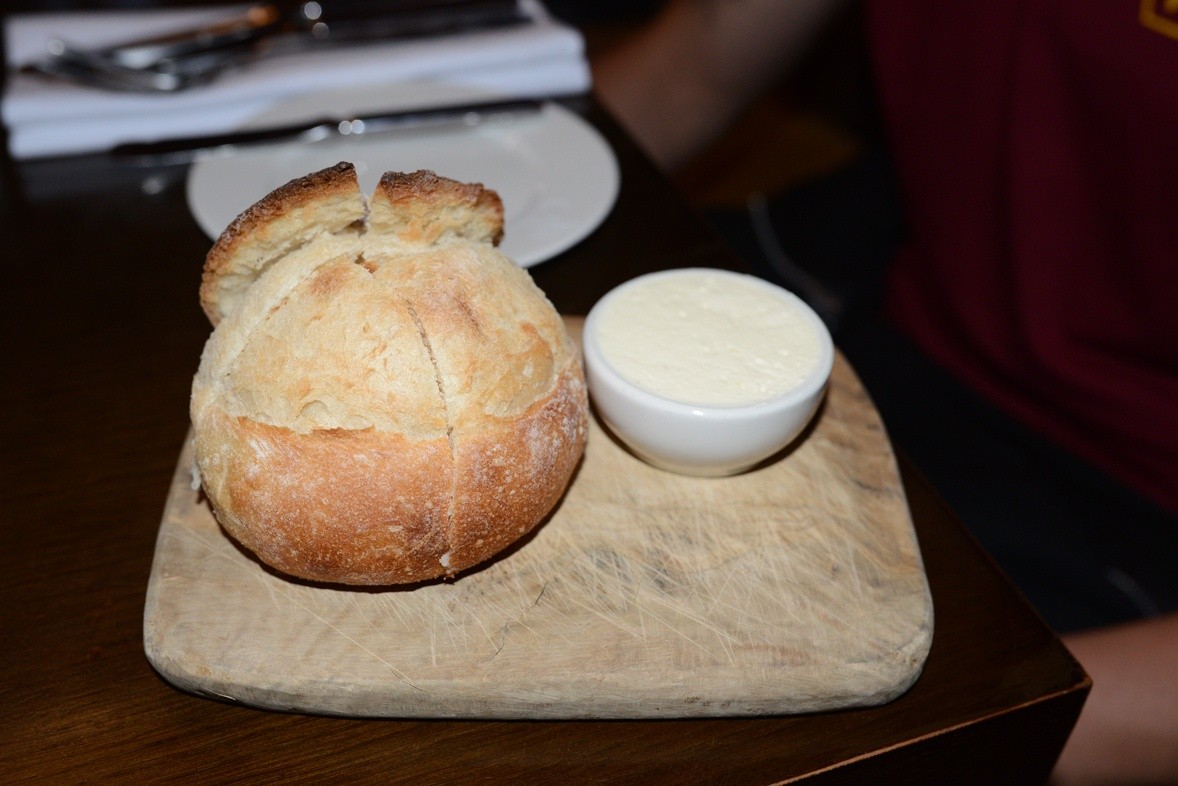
192,161,590,589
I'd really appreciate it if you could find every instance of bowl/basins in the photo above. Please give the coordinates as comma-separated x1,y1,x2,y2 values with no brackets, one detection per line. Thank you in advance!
579,266,837,481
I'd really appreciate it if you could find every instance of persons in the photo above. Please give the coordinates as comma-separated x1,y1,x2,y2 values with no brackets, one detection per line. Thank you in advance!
584,0,1178,786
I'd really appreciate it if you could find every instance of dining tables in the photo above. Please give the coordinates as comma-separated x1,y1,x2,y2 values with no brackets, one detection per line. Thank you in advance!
0,0,1096,786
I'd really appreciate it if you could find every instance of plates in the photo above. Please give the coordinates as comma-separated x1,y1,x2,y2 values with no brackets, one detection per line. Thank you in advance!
178,99,620,275
